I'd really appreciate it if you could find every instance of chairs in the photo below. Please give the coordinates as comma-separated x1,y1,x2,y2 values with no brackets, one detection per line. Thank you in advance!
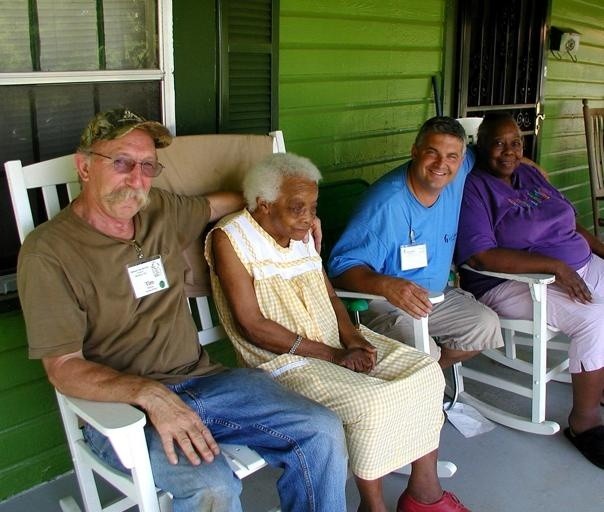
580,97,603,241
313,178,459,417
446,117,574,444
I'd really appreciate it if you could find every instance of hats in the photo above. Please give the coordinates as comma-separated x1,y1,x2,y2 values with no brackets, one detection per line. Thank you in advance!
79,104,175,154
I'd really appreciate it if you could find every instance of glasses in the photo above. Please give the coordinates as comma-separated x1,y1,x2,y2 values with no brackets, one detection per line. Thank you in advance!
87,148,164,179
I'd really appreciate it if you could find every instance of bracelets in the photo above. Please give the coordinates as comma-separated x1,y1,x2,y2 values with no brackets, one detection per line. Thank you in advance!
288,336,304,355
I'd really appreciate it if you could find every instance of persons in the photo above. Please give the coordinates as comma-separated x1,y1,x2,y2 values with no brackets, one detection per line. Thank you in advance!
204,151,470,512
16,107,348,512
459,111,604,468
327,116,503,369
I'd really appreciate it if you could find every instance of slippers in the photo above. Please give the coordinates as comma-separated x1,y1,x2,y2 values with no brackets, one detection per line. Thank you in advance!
561,422,604,472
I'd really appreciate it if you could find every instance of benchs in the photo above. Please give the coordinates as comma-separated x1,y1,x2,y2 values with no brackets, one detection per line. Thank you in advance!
1,128,456,512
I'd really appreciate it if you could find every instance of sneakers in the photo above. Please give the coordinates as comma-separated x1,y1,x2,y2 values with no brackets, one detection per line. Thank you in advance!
394,487,472,512
